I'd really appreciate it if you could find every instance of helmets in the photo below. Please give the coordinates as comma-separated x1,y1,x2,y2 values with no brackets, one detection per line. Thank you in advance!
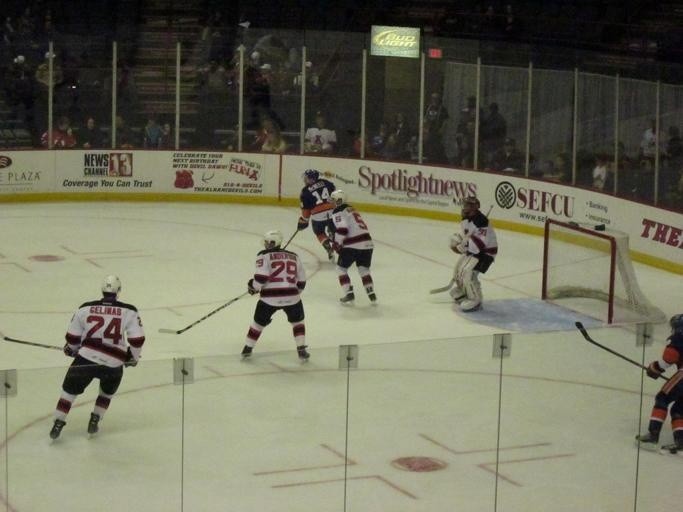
304,167,320,182
330,189,346,205
263,229,283,249
101,272,123,296
460,195,481,212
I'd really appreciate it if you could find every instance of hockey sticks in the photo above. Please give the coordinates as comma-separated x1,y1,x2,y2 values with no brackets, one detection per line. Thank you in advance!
2,335,62,351
159,291,251,334
574,321,668,377
430,204,496,295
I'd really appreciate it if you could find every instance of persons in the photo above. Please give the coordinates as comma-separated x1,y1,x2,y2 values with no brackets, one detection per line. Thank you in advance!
296,168,340,261
240,230,310,359
634,314,683,452
329,189,378,303
0,0,683,213
49,273,146,439
448,196,498,312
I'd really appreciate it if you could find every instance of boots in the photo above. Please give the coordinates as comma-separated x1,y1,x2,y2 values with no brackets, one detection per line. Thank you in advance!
322,240,334,260
450,255,482,311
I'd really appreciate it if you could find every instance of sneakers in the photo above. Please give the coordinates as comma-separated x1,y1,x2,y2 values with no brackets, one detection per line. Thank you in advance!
635,430,661,445
661,436,682,454
85,411,101,435
48,419,67,441
365,287,377,302
338,286,355,302
296,344,311,360
240,344,255,356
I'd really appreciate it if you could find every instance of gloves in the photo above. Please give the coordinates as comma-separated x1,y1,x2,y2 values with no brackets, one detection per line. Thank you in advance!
246,278,261,296
124,346,140,369
450,232,469,254
61,344,81,358
296,215,309,231
644,360,666,380
